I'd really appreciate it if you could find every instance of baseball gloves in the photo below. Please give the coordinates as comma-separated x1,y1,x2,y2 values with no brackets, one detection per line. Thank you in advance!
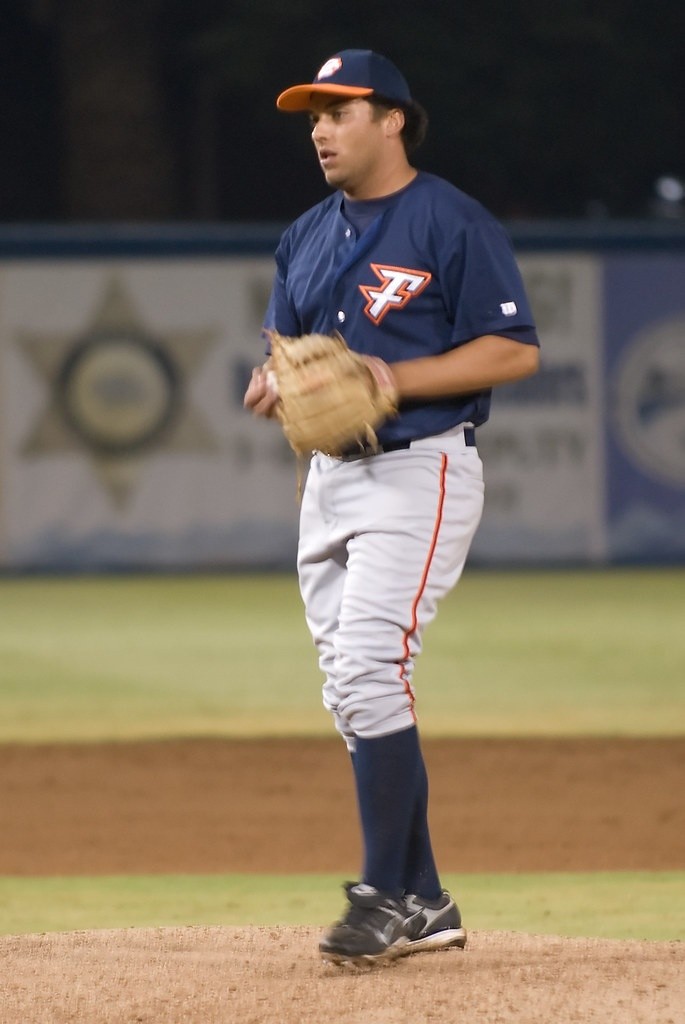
256,325,401,505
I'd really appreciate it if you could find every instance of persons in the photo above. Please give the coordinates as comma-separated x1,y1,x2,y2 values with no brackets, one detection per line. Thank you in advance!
244,50,540,966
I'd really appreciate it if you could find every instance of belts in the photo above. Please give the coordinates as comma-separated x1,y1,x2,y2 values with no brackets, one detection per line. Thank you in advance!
334,425,475,462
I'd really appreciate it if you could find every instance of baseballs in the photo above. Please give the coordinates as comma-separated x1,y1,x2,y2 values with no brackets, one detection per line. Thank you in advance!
258,369,279,393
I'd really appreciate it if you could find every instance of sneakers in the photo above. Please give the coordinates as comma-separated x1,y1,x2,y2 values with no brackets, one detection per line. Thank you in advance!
320,881,466,963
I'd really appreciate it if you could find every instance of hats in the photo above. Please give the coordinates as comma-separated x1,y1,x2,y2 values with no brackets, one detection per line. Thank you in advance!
277,48,413,111
